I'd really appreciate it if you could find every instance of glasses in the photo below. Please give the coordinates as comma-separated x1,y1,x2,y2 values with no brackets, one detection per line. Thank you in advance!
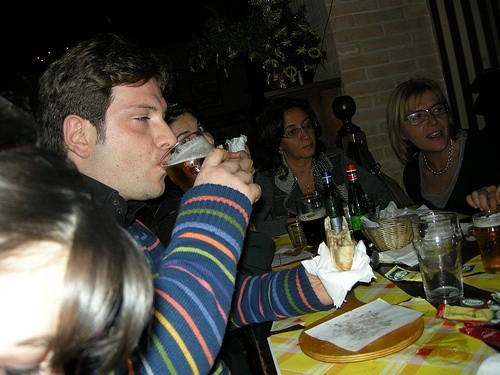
405,102,449,126
282,120,317,139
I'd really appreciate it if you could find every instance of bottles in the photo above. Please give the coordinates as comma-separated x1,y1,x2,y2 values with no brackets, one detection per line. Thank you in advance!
321,170,347,241
345,164,373,248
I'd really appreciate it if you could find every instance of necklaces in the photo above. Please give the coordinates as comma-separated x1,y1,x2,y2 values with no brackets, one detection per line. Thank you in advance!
295,178,312,192
423,142,453,175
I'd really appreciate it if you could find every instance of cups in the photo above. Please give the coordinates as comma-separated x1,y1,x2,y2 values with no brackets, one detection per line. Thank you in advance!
413,211,464,305
286,221,308,248
158,130,218,194
294,193,327,246
472,209,500,272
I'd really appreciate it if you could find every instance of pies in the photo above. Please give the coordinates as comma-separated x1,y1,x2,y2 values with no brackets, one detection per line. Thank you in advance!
324,216,355,271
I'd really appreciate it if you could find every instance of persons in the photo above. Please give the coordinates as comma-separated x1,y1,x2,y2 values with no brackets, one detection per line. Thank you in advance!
386,79,500,216
0,31,358,375
251,96,388,239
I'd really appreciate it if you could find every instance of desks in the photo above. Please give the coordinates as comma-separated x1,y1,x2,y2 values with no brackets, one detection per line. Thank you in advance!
255,204,500,375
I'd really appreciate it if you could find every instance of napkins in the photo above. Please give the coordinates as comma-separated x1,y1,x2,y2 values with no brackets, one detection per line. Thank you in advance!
380,201,435,216
378,241,419,267
305,297,425,352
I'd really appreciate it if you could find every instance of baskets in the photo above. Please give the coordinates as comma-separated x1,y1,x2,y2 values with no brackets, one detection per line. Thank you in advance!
362,213,420,251
